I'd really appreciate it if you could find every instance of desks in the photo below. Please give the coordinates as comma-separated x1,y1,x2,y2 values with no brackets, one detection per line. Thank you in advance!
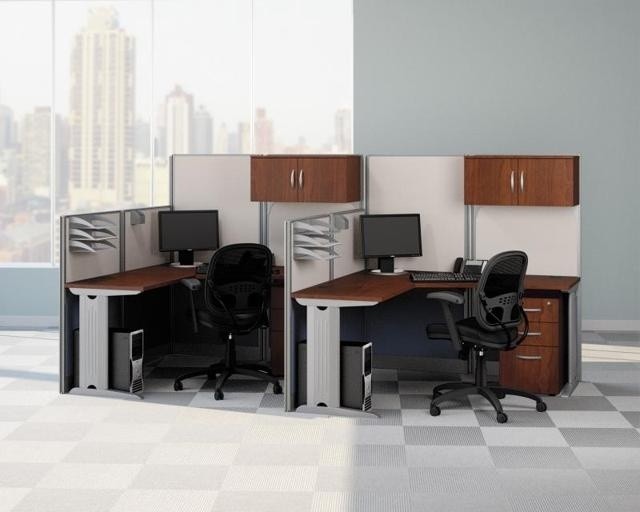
65,261,284,400
289,269,581,419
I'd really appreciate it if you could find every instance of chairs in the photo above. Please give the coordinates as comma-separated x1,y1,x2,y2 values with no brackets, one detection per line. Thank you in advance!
174,243,282,401
425,250,546,423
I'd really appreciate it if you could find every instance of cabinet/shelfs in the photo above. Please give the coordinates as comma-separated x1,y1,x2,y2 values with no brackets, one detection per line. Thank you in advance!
464,155,580,206
250,154,361,203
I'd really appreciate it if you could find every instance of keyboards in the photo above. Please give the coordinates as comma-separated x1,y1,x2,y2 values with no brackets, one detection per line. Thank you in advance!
196,264,208,274
409,271,483,282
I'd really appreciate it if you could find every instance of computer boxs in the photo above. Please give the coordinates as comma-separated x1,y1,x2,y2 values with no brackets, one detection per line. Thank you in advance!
71,328,144,394
296,340,372,413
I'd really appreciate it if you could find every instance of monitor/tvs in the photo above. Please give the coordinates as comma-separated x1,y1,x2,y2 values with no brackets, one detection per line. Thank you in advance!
158,210,220,268
361,214,423,276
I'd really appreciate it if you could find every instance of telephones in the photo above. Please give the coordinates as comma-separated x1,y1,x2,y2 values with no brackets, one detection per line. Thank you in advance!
453,258,488,276
